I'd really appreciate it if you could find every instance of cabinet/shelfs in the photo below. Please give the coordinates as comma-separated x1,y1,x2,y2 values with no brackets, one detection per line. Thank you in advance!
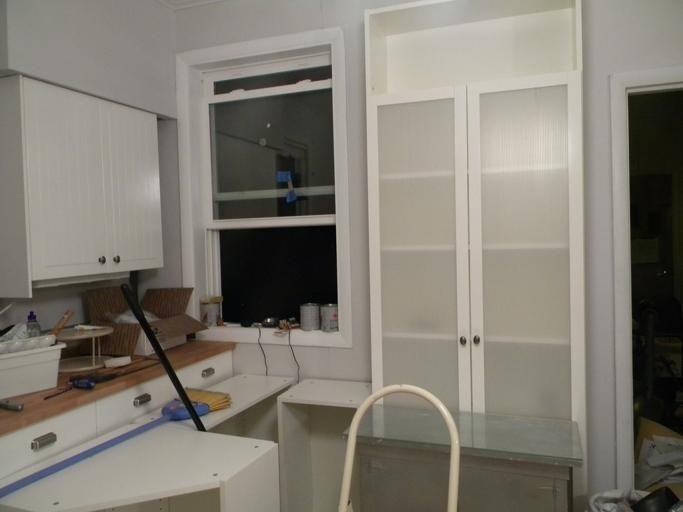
0,340,237,479
0,75,164,297
133,375,299,433
0,425,279,511
272,379,374,511
362,1,589,512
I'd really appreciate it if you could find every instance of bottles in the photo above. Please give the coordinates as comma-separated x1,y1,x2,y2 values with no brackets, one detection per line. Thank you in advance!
26,311,41,337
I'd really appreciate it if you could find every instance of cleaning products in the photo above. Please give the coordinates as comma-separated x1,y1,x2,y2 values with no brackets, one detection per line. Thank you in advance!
27,308,44,338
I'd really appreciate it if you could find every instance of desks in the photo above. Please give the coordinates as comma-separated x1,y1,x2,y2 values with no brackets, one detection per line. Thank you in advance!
341,409,587,510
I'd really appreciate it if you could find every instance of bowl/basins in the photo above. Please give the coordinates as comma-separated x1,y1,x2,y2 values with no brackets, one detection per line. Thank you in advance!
261,317,278,327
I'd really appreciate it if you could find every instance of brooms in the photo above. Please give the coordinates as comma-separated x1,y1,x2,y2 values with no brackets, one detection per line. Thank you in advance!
0,386,233,498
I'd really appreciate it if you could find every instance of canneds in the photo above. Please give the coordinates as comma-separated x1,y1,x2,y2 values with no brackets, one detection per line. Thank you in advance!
200,296,223,327
300,302,320,331
319,304,339,332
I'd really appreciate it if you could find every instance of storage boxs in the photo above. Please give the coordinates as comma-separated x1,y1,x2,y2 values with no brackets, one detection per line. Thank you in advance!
86,284,207,358
0,339,70,398
